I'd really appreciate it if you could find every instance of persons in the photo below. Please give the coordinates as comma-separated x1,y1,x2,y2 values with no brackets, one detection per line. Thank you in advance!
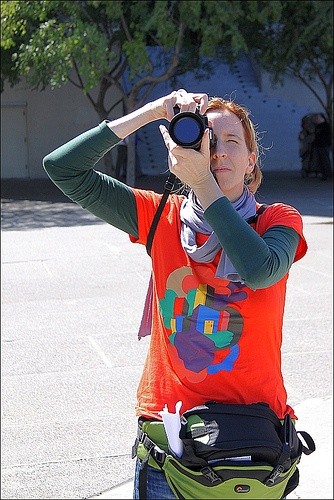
42,89,308,500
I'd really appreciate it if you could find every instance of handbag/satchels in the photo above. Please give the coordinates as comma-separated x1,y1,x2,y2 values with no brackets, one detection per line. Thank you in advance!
135,401,316,498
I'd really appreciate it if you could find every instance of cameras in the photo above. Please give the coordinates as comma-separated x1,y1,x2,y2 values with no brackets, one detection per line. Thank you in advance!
169,106,218,152
